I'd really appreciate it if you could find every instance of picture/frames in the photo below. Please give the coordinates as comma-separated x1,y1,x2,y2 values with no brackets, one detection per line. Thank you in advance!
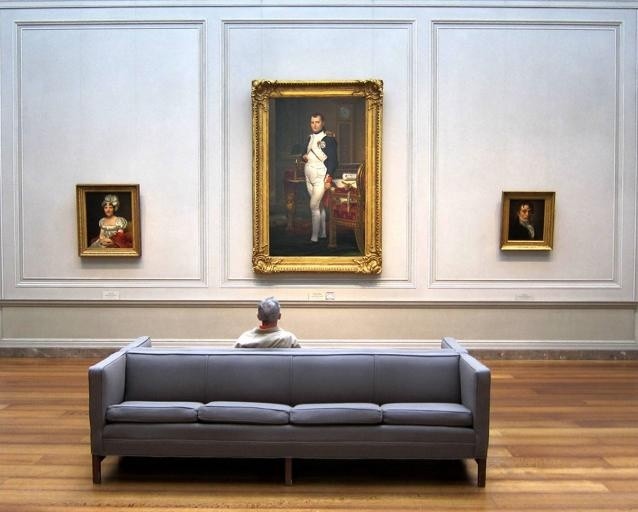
77,184,143,258
499,189,556,253
250,78,383,275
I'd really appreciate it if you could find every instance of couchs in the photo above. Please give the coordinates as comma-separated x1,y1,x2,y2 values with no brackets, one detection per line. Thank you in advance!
130,336,467,354
91,337,490,488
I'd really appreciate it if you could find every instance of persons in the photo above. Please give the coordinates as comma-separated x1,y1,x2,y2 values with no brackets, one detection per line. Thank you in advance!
232,297,303,348
511,201,541,239
89,195,127,248
301,114,338,245
